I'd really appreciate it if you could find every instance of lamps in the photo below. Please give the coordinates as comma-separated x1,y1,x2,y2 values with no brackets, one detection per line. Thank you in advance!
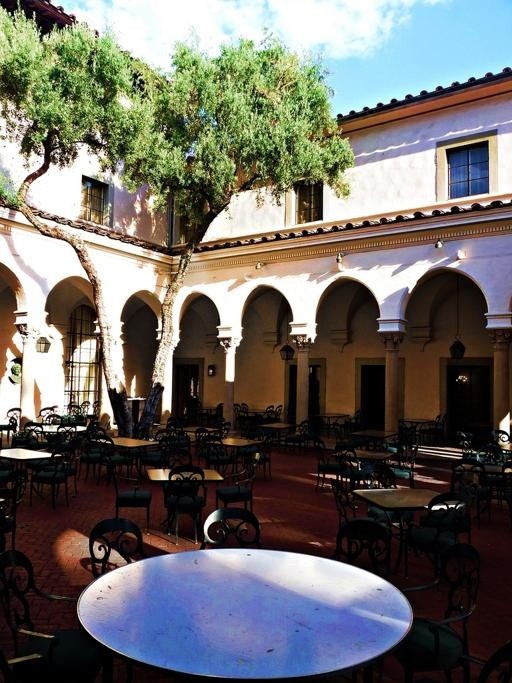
433,237,446,249
449,274,465,359
255,259,267,269
278,304,295,362
335,250,348,263
